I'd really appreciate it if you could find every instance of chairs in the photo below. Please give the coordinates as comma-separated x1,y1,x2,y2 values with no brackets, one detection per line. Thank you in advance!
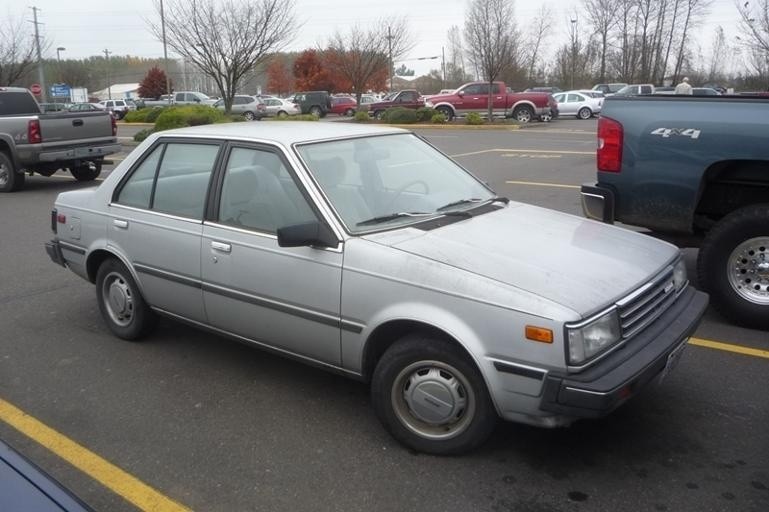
226,170,286,232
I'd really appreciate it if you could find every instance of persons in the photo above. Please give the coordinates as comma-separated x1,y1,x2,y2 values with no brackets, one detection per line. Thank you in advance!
674,76,693,95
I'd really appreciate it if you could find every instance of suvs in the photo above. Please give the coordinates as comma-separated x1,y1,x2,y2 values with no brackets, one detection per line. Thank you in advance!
211,94,266,121
291,91,331,118
97,99,129,121
366,90,425,122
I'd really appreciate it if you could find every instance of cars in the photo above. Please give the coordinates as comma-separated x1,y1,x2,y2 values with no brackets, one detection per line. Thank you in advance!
210,95,221,100
42,120,709,457
67,102,116,120
328,96,358,117
521,82,731,124
333,92,385,111
124,94,173,112
39,102,68,115
253,94,306,103
261,98,302,119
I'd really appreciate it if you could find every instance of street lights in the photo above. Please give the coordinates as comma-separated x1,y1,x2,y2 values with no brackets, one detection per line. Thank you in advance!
195,41,208,92
56,46,68,84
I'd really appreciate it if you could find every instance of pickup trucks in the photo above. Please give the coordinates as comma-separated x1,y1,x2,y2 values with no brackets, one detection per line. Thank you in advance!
423,82,555,123
143,91,218,109
0,87,119,194
579,87,768,332
